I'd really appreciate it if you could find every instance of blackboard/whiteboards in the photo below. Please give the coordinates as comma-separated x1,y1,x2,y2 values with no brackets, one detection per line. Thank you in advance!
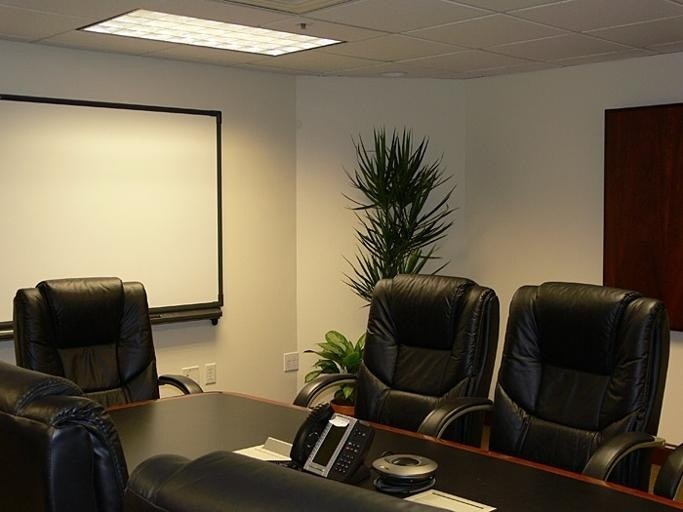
0,93,223,339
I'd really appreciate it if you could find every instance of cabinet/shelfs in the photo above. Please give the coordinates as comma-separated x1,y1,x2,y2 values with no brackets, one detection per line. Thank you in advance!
602,103,683,333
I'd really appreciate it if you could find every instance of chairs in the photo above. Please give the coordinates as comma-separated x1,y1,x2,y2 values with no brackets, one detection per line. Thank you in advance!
416,274,676,492
290,273,503,455
2,360,131,511
114,447,458,512
10,275,205,398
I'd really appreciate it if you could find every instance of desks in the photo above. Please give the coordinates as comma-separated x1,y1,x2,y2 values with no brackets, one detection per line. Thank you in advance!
51,387,683,512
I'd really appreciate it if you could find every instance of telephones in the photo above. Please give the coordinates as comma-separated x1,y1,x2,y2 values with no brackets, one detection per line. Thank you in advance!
289,402,375,482
